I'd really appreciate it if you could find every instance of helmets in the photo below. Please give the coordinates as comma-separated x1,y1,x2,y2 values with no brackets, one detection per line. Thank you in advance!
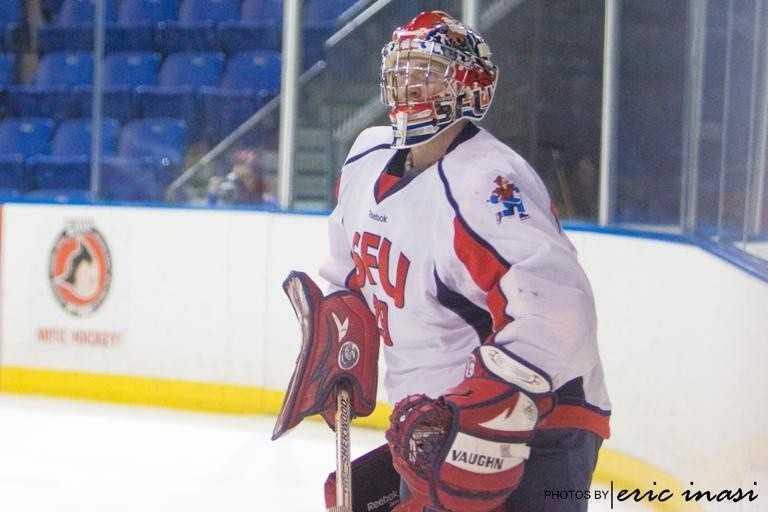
378,6,502,153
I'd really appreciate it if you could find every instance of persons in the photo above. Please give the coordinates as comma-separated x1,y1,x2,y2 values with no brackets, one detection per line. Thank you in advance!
209,149,278,205
315,13,610,512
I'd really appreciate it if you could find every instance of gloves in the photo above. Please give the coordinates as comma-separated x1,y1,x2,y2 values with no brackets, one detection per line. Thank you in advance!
381,338,558,512
269,268,384,445
